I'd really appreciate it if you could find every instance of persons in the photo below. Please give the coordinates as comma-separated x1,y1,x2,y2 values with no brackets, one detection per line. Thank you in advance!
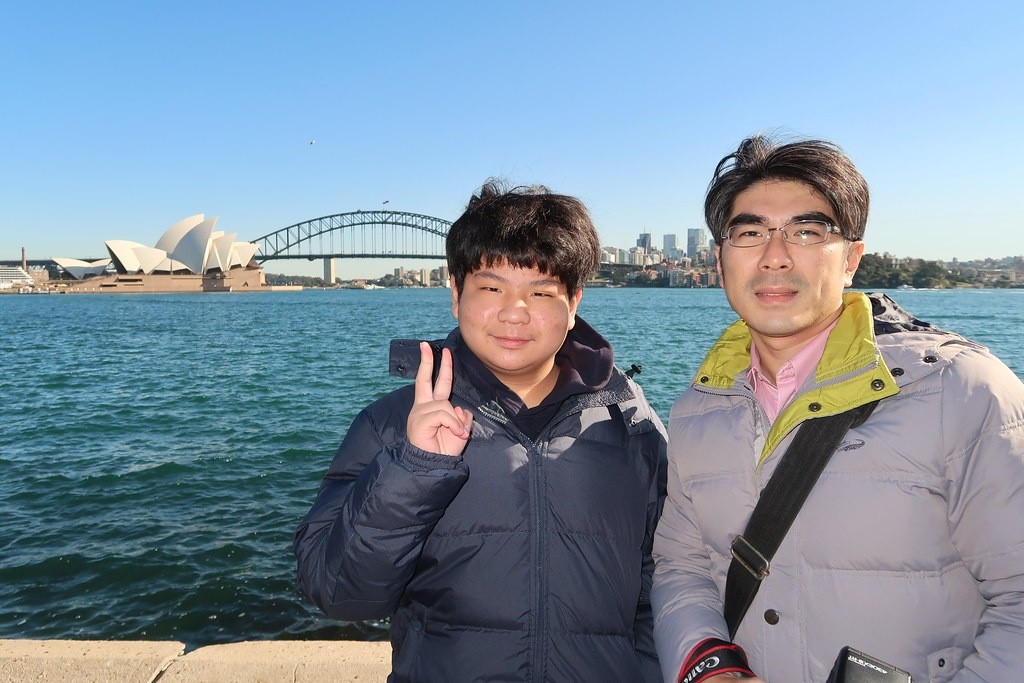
651,135,1024,683
294,176,669,683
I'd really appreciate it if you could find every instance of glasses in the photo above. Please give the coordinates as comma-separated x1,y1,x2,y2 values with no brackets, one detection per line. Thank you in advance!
715,217,840,249
